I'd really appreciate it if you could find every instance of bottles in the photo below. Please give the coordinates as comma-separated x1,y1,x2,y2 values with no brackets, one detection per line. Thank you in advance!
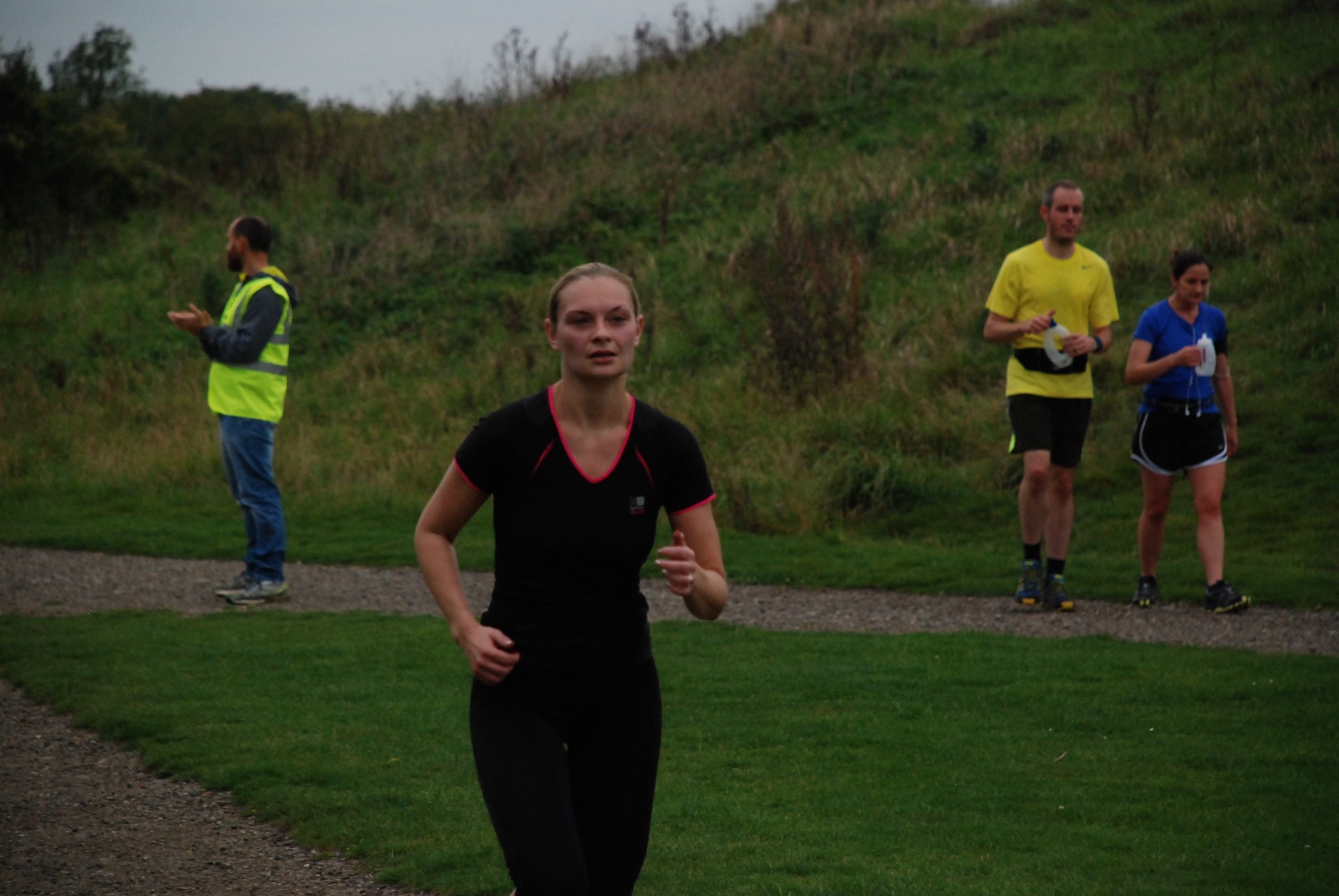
1043,319,1073,369
1195,332,1217,377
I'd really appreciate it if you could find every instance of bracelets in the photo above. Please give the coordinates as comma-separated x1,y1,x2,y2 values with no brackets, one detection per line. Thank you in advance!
1092,335,1103,353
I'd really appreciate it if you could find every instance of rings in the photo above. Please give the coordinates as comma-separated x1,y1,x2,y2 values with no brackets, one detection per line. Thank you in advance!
688,575,693,582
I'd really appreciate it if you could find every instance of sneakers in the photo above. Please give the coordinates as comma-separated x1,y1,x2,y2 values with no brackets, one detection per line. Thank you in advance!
1016,556,1043,604
1205,582,1252,614
1042,574,1075,611
1137,574,1161,609
214,572,292,605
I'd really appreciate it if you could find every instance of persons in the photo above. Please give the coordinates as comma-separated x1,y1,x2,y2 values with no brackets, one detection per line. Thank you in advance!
1124,248,1251,613
167,215,296,607
983,180,1120,613
414,261,730,896
